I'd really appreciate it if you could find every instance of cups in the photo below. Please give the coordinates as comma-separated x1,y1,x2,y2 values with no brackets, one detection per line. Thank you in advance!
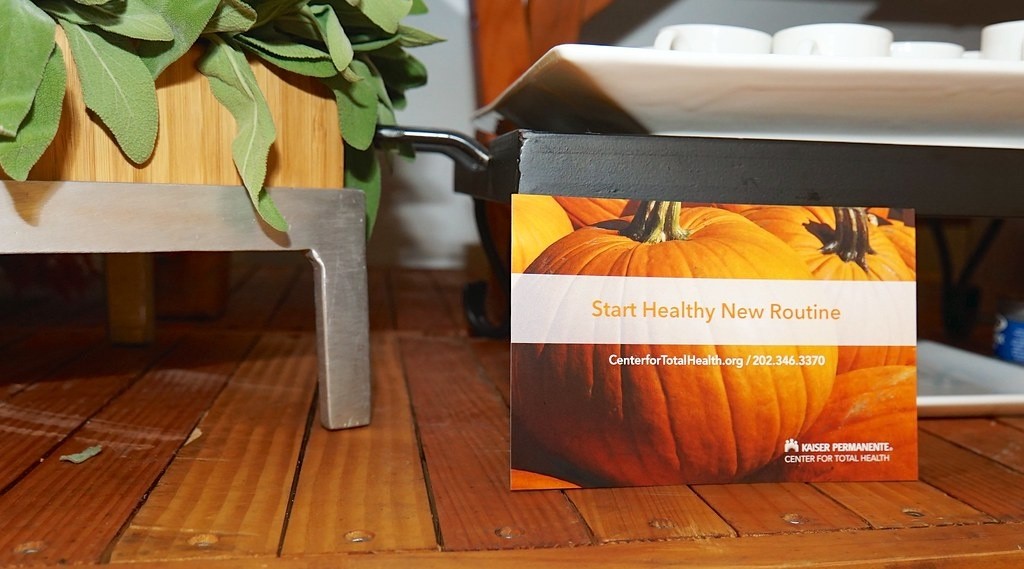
769,21,892,58
888,42,964,61
981,18,1023,64
656,23,773,54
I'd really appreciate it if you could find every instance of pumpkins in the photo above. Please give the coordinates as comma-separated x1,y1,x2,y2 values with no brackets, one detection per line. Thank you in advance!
510,194,918,490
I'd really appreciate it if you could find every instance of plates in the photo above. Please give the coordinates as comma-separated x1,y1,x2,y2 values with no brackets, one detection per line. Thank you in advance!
915,336,1024,419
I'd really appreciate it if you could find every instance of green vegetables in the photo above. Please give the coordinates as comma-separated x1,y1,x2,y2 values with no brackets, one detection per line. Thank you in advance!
0,0,449,249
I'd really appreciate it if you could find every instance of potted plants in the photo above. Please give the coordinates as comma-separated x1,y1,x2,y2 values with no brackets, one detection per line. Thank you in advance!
2,0,447,248
